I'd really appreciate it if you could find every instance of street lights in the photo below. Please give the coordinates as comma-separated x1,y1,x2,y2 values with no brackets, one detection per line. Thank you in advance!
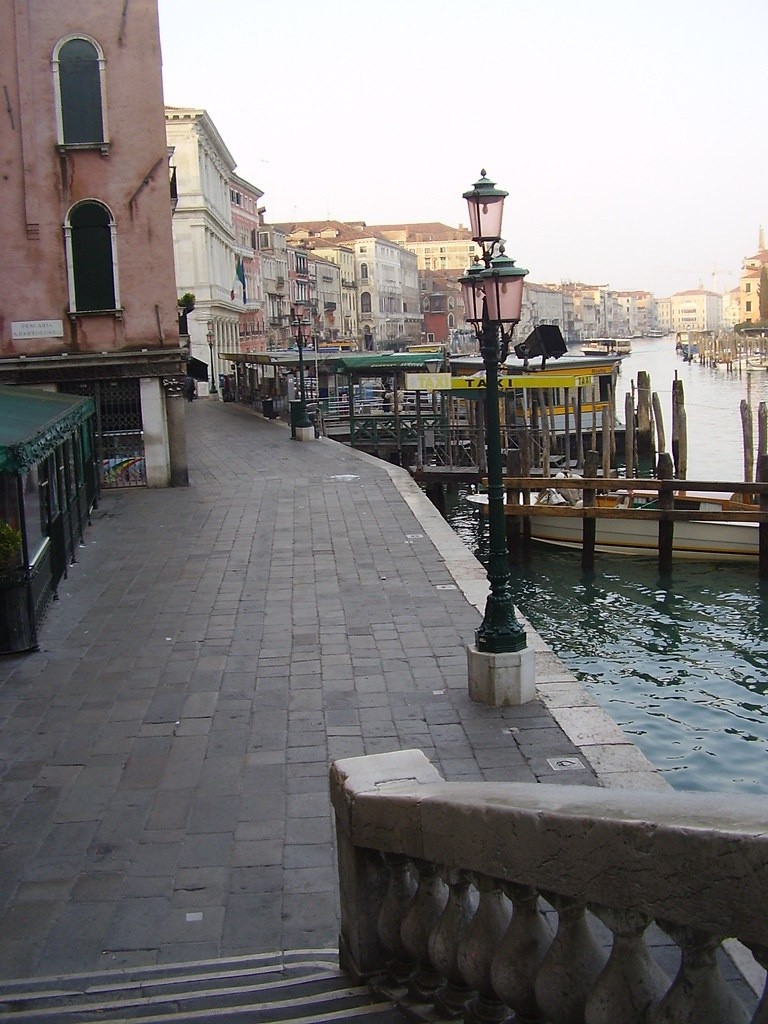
207,319,219,394
454,166,527,653
290,298,314,427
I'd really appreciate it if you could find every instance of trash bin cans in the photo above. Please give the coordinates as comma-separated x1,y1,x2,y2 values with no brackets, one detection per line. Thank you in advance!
288,399,302,441
262,398,273,417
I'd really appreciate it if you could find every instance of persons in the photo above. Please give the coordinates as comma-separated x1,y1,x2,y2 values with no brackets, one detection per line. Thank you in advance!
184,376,195,402
382,383,393,412
384,385,404,412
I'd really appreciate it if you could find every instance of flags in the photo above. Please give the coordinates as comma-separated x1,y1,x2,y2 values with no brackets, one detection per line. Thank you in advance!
235,256,247,305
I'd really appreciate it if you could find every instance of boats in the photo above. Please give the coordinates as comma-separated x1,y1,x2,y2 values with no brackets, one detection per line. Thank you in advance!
580,338,632,357
644,329,663,338
219,337,767,562
